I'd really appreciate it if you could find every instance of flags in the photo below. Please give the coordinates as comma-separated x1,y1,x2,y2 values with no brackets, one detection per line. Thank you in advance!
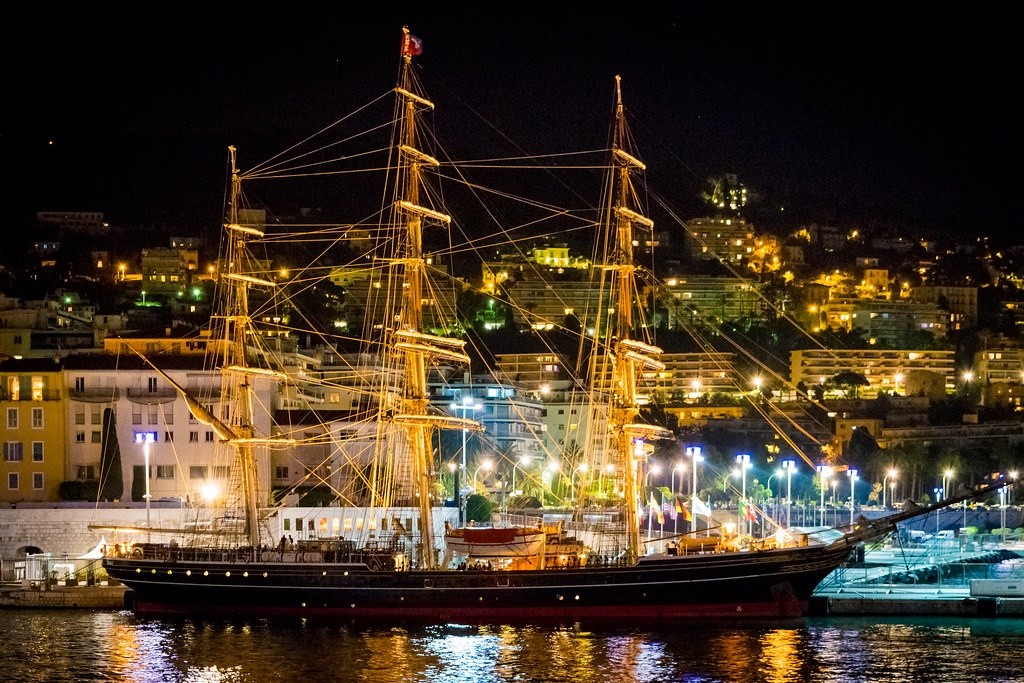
662,497,670,513
650,497,664,523
738,501,758,523
637,500,643,525
676,498,692,521
403,35,422,55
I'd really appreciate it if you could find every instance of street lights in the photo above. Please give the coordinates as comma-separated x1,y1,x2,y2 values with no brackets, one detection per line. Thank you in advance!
781,460,796,528
737,454,751,501
513,458,529,495
686,447,702,539
768,470,781,499
882,468,896,507
542,464,555,499
943,469,953,500
473,461,491,494
571,464,584,499
671,464,685,502
724,469,740,493
598,465,612,492
846,470,859,530
645,463,657,486
133,431,155,543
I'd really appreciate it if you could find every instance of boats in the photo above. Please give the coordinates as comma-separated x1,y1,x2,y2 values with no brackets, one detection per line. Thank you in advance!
443,521,543,555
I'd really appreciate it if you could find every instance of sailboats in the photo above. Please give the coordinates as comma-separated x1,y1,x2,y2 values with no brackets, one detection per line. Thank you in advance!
55,24,1024,621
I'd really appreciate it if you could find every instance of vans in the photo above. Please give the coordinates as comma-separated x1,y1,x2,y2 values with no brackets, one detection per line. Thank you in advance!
937,530,955,539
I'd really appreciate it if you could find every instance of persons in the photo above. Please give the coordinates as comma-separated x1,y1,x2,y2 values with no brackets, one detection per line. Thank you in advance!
281,535,286,548
289,535,293,544
457,560,491,570
